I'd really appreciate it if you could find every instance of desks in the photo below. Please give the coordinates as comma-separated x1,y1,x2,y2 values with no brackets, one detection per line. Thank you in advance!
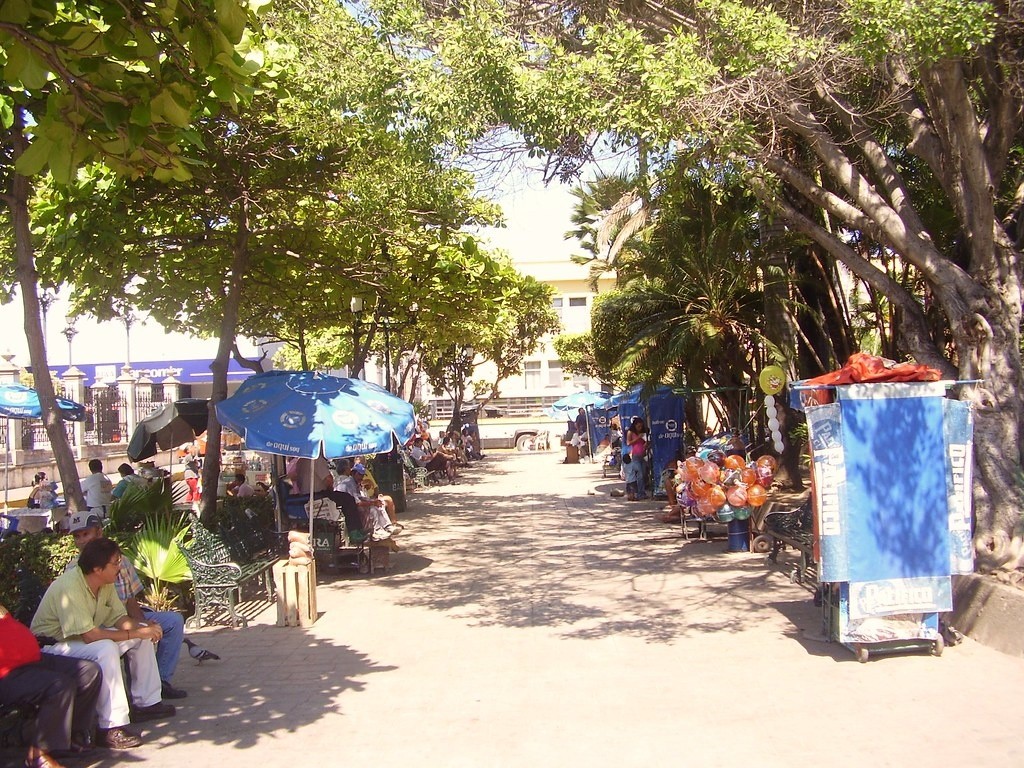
272,557,319,629
0,508,52,543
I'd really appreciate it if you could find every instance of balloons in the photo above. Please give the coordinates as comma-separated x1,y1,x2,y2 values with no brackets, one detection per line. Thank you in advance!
759,365,785,395
765,395,784,454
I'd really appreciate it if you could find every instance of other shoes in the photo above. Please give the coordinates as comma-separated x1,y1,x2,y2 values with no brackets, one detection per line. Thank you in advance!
628,497,639,501
95,723,142,748
23,753,69,768
71,741,92,754
449,480,461,485
385,525,402,535
161,681,187,699
371,529,391,542
131,701,175,722
480,454,485,460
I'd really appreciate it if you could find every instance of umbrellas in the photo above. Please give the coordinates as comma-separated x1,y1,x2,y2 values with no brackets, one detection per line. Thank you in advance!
0,382,86,503
548,391,608,421
214,370,417,541
603,382,674,411
126,398,210,476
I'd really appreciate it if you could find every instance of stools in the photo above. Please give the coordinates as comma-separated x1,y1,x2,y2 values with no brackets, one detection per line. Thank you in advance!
364,538,399,574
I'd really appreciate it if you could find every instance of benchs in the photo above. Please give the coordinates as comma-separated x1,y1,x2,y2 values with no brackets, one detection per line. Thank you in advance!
0,604,155,745
175,513,290,628
403,450,439,488
764,491,813,584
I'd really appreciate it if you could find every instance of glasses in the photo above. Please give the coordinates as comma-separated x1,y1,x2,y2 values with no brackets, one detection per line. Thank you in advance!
108,560,121,567
44,478,48,480
254,489,262,492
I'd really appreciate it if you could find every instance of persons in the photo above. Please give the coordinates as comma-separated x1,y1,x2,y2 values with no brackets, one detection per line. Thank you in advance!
0,602,103,768
80,458,112,507
26,472,59,508
576,408,587,436
110,463,138,501
286,455,404,541
412,438,455,485
183,453,202,502
612,424,622,443
65,508,189,699
623,418,647,502
438,430,485,469
29,537,175,749
235,473,254,498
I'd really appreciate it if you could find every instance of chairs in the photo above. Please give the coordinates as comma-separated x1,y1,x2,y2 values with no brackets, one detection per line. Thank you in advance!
680,506,707,540
280,476,318,518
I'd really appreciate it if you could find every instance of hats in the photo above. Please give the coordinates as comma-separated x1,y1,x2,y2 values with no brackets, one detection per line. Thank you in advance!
351,463,368,476
67,511,101,535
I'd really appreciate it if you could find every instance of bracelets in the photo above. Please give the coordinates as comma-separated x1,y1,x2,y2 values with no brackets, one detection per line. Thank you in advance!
127,630,129,640
